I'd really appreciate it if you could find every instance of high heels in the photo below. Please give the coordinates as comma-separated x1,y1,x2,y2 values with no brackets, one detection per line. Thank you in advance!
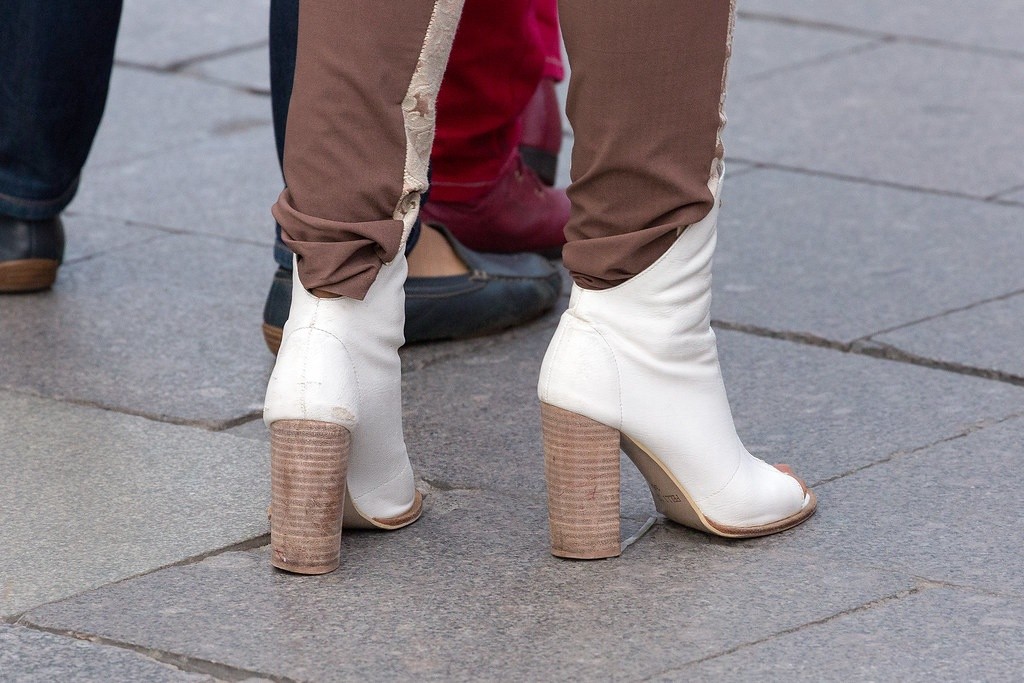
263,251,424,576
535,160,817,558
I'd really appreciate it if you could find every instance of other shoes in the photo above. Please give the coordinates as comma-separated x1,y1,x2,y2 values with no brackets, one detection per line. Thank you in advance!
417,155,573,254
0,215,64,292
516,80,563,187
262,220,562,356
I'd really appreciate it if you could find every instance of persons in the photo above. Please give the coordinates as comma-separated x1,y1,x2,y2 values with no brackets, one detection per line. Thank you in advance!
265,0,817,575
416,1,572,261
0,0,561,360
